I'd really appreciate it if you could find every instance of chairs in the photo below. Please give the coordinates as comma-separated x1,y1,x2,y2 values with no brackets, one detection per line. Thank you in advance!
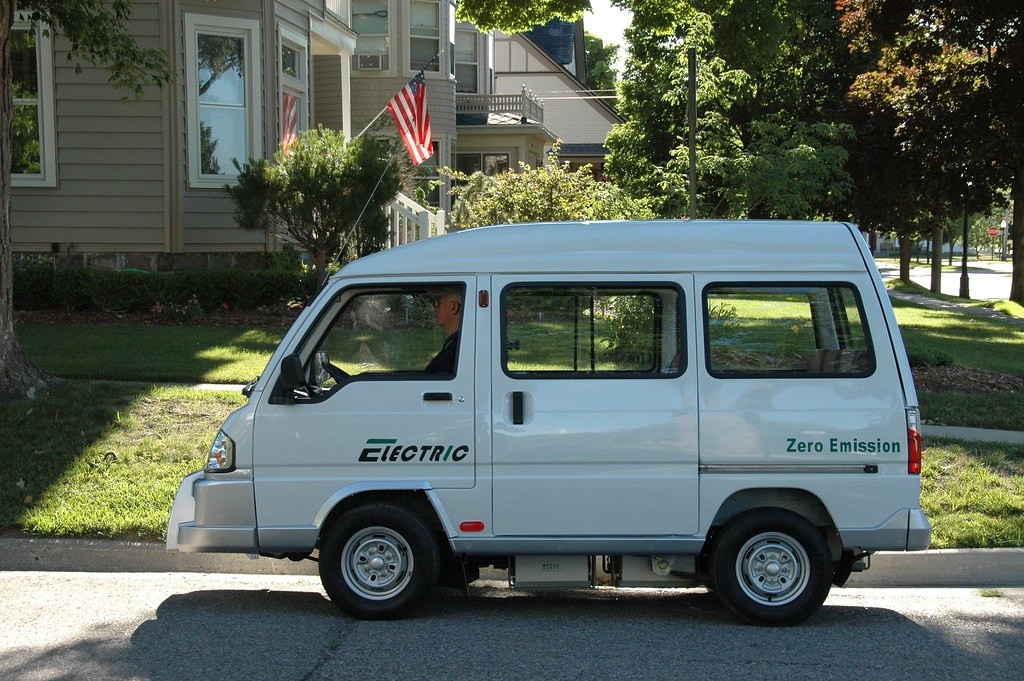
808,348,868,373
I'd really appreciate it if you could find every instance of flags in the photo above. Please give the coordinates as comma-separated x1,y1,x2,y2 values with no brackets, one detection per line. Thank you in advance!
281,92,298,152
386,55,439,168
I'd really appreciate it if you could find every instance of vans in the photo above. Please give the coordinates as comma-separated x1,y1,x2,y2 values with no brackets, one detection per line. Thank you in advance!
159,210,931,628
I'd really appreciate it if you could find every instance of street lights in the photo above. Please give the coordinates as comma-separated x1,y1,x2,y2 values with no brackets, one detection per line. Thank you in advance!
1000,219,1007,261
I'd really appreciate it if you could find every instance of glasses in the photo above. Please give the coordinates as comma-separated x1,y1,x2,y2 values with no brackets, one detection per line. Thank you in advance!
431,298,461,310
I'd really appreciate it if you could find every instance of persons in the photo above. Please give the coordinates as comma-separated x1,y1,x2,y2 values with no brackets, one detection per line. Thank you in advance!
423,285,462,372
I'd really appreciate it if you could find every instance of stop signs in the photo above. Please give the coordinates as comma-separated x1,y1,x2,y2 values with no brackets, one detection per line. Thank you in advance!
988,226,998,237
